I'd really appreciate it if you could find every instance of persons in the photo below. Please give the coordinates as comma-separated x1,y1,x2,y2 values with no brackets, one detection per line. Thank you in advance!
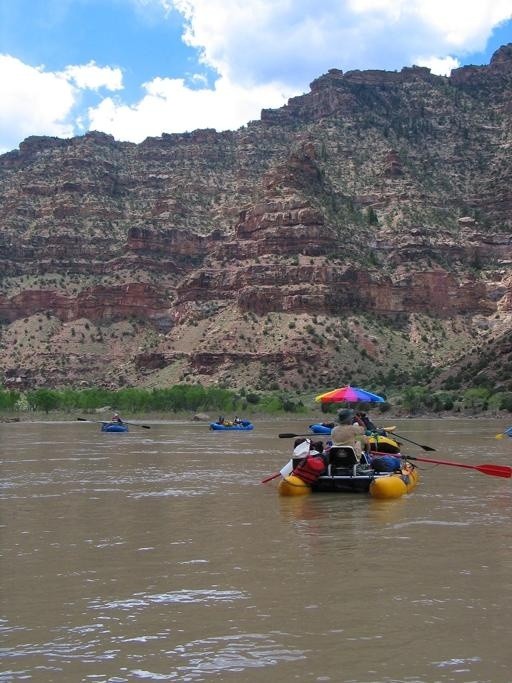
112,414,122,423
331,408,367,462
234,415,239,424
322,417,337,428
352,412,376,430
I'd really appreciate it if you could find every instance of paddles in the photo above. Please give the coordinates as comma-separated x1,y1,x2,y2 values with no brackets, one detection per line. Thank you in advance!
371,451,511,478
78,418,105,424
122,422,149,429
278,433,331,437
495,428,511,439
388,433,437,451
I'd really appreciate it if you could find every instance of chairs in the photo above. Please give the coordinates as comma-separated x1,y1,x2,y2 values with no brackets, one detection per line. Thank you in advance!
328,445,360,477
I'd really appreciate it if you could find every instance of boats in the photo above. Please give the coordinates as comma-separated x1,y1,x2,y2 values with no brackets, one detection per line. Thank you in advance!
277,423,418,499
210,422,254,431
102,422,127,433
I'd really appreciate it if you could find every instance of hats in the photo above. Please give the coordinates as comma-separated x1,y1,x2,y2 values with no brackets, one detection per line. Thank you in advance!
336,410,354,423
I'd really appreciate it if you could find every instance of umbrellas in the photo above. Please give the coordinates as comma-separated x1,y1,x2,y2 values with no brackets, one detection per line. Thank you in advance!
316,385,384,410
